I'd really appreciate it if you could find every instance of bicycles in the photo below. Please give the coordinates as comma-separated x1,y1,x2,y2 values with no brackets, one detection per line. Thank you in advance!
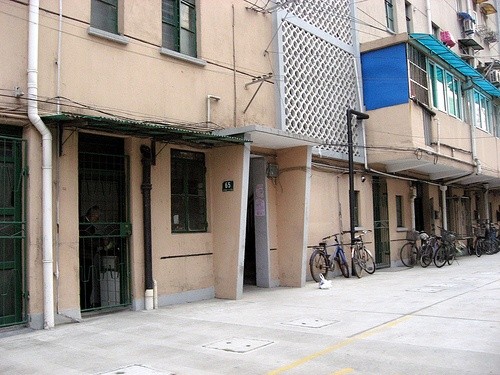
351,229,375,278
309,232,349,284
399,217,500,269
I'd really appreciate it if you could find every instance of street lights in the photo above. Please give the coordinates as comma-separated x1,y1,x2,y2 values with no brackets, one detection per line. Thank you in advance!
346,107,369,277
